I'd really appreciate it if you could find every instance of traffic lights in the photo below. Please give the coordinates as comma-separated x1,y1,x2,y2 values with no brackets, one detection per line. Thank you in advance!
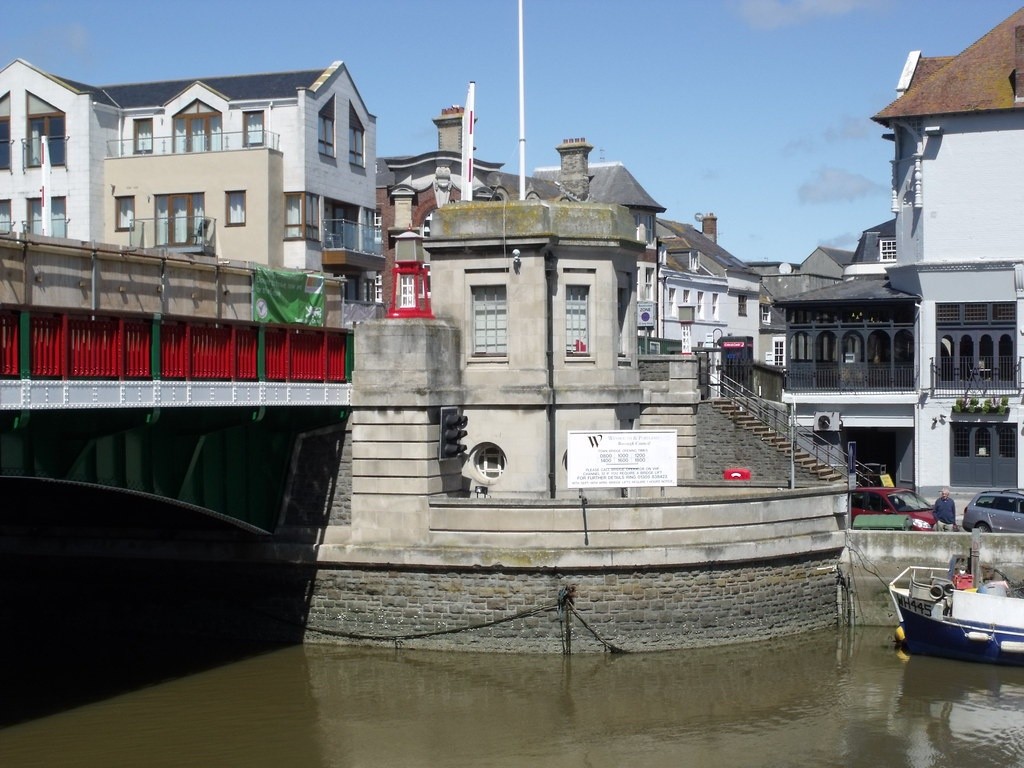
442,408,468,464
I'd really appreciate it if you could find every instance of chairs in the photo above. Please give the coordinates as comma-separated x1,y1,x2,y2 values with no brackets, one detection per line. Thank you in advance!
190,218,211,245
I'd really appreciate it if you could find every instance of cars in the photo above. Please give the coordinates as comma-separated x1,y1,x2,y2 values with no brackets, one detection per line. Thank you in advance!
849,486,957,532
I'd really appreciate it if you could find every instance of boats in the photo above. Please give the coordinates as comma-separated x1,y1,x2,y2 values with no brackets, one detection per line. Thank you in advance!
889,529,1024,665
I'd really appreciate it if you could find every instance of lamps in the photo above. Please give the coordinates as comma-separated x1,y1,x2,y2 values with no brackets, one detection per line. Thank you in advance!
512,248,522,267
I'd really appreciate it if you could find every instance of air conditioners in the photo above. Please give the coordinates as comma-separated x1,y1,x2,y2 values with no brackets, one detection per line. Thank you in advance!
813,411,841,432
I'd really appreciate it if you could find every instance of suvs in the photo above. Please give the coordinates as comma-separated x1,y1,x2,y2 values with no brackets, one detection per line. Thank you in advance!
963,491,1024,533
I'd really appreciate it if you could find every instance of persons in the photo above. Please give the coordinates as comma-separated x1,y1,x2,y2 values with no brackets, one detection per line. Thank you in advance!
933,489,957,532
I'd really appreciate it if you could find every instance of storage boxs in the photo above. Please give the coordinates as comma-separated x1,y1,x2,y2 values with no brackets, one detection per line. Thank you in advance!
954,575,973,589
724,469,750,481
